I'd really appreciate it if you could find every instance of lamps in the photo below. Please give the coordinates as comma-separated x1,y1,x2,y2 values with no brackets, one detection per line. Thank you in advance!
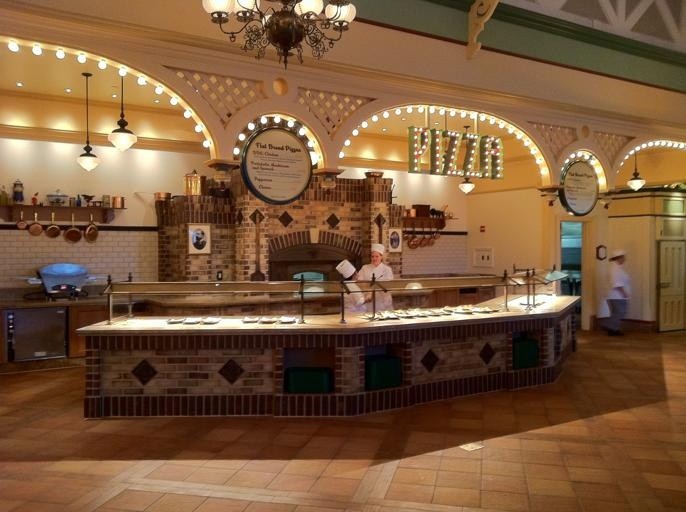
107,74,137,150
76,72,100,171
201,0,356,71
626,152,646,190
458,125,476,195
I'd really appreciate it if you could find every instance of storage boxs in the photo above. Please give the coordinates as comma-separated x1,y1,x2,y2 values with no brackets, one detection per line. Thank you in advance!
412,204,431,217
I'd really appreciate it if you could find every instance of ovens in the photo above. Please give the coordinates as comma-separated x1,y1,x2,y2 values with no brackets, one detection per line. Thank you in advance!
0,307,66,364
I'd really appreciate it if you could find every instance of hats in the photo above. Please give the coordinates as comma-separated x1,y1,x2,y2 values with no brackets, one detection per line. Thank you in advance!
371,243,385,256
609,250,625,259
336,259,356,279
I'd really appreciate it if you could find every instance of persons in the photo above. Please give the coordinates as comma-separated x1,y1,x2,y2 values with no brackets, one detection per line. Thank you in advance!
342,269,373,314
357,252,395,313
599,254,631,337
194,229,206,249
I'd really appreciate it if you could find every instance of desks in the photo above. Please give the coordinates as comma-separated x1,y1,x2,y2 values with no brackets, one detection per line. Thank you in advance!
0,267,581,421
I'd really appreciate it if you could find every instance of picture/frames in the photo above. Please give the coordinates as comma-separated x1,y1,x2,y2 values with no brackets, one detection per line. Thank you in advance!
186,223,212,255
471,247,495,267
388,227,403,254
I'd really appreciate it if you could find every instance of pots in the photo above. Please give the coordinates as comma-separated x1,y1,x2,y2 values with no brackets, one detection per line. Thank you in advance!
403,222,442,249
46,188,68,206
16,207,98,243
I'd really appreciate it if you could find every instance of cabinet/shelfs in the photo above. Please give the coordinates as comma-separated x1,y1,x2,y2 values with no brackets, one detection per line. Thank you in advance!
69,277,497,357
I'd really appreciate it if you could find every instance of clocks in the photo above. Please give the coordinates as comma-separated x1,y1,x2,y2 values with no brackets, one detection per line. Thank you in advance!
596,244,607,260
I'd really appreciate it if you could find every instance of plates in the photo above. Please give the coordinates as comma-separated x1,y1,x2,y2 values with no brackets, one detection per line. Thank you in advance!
376,308,499,320
243,316,296,324
169,317,221,324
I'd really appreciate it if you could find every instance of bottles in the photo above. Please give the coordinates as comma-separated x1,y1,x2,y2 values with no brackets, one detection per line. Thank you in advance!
70,197,75,207
